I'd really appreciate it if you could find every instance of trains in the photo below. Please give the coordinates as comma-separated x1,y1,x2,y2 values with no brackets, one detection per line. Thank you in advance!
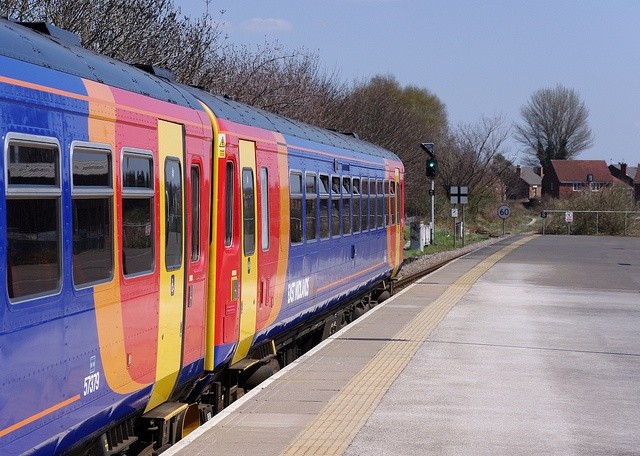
0,16,405,456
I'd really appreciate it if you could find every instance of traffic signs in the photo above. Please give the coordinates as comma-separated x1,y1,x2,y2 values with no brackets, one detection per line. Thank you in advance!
497,205,510,219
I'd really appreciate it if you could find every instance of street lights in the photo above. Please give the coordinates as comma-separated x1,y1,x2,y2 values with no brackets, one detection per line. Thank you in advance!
587,173,593,199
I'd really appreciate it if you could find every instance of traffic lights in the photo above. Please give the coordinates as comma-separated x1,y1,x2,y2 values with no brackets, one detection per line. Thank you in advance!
426,160,437,177
